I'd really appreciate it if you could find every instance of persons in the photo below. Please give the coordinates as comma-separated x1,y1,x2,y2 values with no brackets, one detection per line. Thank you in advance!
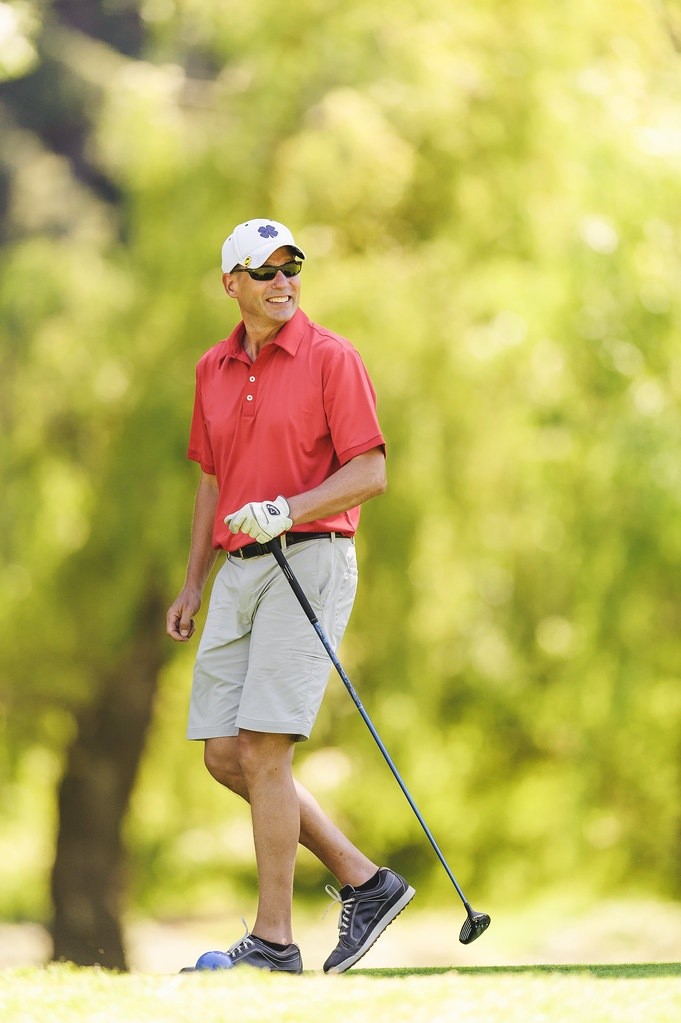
166,220,417,976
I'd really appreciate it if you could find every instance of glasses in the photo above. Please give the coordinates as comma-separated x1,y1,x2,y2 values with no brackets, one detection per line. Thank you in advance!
228,261,304,282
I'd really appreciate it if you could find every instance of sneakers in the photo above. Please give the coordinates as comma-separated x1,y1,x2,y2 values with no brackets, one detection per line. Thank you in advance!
180,918,304,977
323,867,415,973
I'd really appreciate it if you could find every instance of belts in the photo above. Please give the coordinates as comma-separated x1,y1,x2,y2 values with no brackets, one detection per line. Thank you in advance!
230,531,352,560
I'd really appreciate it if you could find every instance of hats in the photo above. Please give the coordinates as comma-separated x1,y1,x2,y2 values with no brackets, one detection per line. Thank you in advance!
220,218,306,275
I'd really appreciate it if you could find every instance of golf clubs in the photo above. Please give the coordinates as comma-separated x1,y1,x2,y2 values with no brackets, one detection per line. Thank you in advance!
268,540,491,946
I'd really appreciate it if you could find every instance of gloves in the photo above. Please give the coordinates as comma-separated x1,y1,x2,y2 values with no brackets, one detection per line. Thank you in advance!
223,496,294,544
